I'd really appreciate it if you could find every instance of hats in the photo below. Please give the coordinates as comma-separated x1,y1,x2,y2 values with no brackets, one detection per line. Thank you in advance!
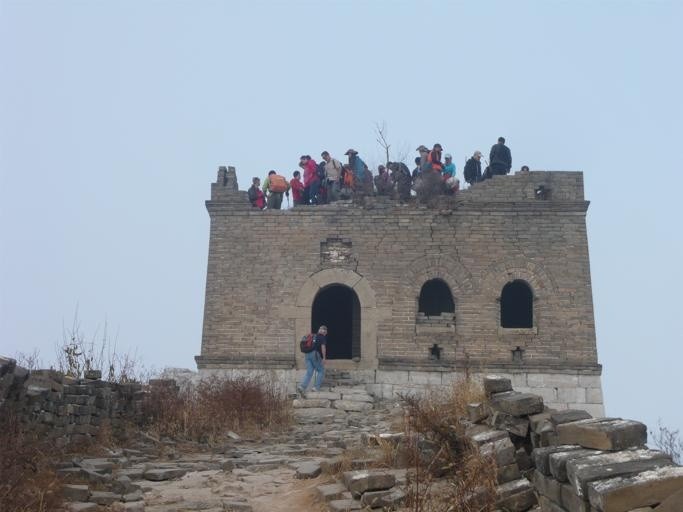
320,326,328,330
344,149,358,155
416,145,428,151
434,144,443,152
445,153,452,159
474,151,484,157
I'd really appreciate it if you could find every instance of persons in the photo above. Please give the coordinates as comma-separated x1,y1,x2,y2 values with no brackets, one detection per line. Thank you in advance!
248,136,529,213
297,325,327,395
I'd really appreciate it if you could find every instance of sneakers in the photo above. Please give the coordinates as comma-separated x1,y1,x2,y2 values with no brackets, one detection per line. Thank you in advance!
311,388,317,392
298,386,306,399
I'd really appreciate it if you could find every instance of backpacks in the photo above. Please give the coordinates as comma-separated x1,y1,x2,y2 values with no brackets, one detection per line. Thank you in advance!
270,175,288,192
300,334,317,353
